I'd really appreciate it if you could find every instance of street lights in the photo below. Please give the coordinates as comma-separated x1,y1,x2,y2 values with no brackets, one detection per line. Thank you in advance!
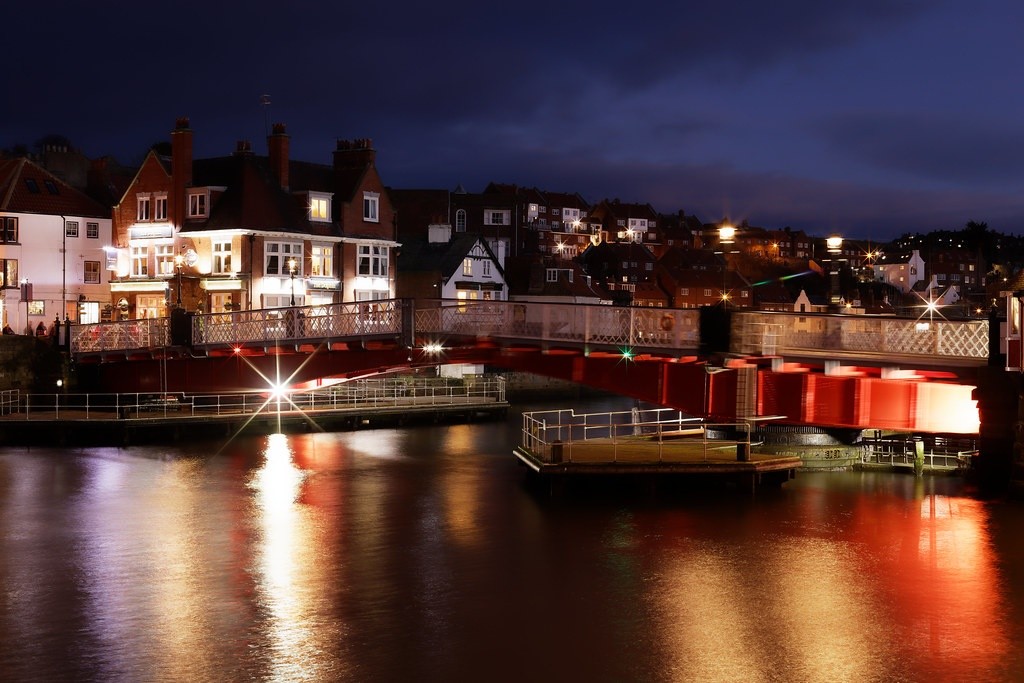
173,251,186,308
286,257,306,307
